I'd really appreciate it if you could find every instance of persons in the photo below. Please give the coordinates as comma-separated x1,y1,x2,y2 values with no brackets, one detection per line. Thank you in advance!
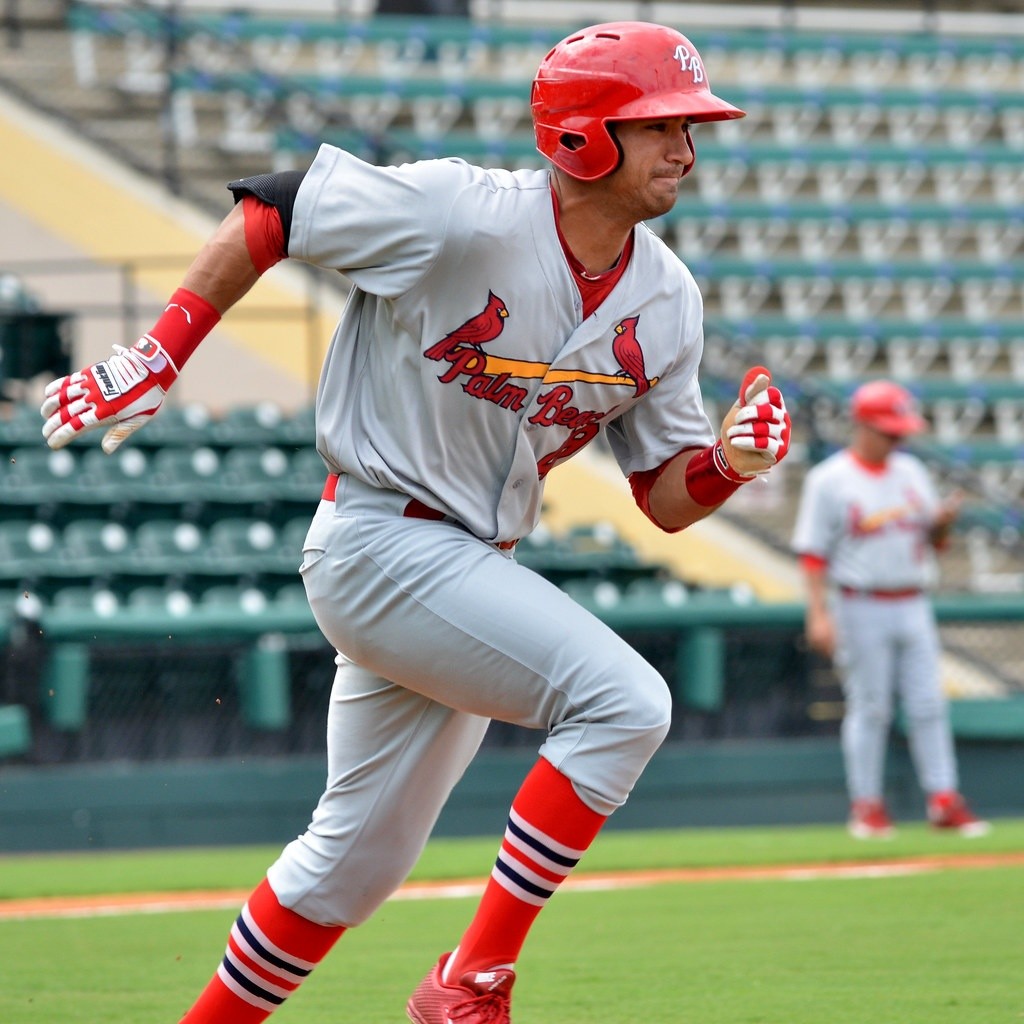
40,20,793,1024
792,380,987,839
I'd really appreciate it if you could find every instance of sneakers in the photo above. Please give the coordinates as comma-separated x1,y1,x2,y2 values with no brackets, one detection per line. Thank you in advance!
847,802,896,843
927,792,991,838
407,952,516,1024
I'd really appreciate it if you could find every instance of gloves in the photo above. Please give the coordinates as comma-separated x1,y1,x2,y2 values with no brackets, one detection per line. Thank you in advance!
685,365,792,508
41,286,221,456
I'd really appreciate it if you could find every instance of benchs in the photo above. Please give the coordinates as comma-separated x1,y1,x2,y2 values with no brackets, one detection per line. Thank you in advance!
1,302,802,737
73,0,1023,571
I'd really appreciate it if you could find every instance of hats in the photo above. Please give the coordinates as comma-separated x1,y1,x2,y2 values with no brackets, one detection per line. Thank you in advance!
853,382,927,438
528,21,747,184
